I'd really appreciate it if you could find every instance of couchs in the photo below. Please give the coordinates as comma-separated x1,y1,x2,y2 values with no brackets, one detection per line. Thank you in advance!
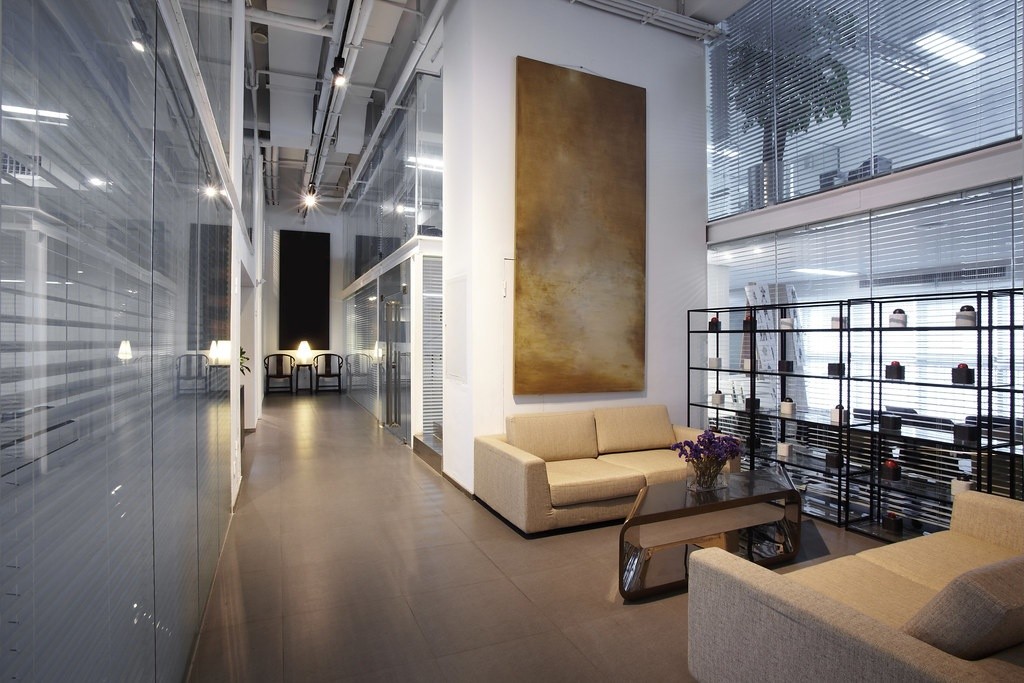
471,401,742,535
685,490,1024,683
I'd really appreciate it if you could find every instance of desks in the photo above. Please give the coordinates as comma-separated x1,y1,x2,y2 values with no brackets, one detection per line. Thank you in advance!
295,360,312,396
209,364,230,395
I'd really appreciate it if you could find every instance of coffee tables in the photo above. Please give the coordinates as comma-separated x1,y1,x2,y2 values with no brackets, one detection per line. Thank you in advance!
619,465,801,602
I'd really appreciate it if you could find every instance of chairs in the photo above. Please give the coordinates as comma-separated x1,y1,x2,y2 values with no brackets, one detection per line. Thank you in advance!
846,154,891,180
345,354,375,395
264,353,296,398
311,354,343,393
174,354,209,397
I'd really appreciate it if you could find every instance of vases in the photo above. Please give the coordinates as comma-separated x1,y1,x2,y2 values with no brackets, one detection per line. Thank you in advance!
685,460,727,492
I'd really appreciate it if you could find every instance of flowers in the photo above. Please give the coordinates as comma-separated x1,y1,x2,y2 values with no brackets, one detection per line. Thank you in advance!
670,430,740,485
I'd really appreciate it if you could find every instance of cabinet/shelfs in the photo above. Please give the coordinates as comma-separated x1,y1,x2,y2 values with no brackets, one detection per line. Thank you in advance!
749,301,876,527
845,292,1016,543
987,287,1024,503
688,288,784,470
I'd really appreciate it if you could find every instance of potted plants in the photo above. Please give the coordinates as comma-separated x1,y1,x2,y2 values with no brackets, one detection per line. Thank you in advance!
732,5,857,212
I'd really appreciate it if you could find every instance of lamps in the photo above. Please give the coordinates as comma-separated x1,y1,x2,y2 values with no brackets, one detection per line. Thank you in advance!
306,182,317,206
118,339,133,360
331,66,344,85
